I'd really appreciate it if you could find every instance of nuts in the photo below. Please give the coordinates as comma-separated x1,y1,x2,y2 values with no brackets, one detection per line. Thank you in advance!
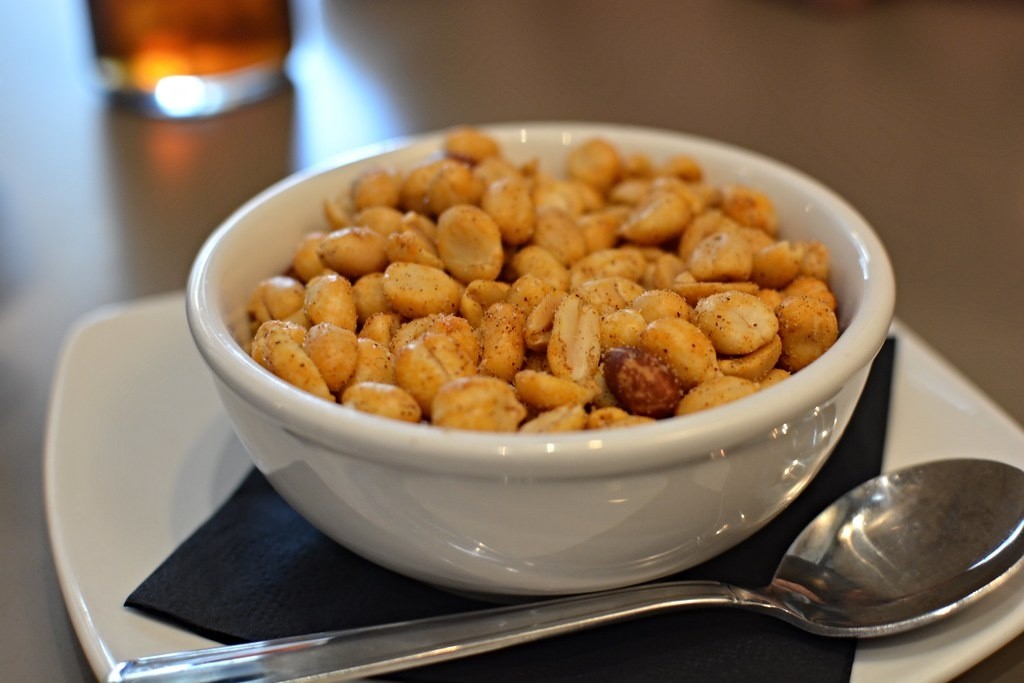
239,131,838,435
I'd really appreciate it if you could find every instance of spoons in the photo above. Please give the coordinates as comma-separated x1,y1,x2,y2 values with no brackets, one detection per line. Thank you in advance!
105,456,1024,683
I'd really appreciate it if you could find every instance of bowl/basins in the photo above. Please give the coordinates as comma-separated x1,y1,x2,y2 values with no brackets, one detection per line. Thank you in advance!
184,119,898,599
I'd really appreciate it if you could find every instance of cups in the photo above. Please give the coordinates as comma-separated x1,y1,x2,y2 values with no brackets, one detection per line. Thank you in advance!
88,0,296,122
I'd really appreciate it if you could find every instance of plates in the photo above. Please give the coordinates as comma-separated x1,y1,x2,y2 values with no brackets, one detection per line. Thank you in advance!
38,286,1024,683
